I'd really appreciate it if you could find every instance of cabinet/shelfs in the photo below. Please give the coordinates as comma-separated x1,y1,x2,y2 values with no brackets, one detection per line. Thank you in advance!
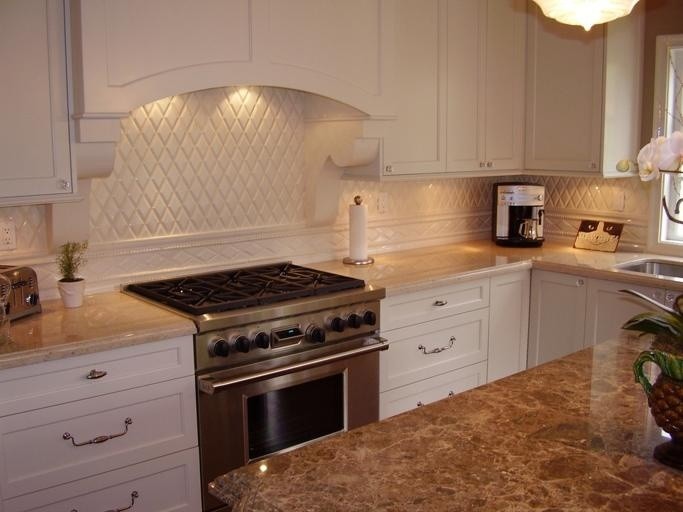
526,269,683,369
380,267,530,420
0,0,85,208
0,335,206,512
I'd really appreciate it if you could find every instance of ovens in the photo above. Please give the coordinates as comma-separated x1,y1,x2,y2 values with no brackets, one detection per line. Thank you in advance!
196,331,391,511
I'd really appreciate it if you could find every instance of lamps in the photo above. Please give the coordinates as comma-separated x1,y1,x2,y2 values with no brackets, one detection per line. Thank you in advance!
534,0,640,31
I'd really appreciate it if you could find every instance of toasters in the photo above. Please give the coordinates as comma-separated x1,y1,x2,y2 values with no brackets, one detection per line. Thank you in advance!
0,265,42,320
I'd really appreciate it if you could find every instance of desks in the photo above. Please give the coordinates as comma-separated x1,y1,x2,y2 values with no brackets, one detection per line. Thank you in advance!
208,331,683,512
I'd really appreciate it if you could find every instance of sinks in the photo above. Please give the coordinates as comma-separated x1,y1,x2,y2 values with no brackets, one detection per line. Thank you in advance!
612,256,682,283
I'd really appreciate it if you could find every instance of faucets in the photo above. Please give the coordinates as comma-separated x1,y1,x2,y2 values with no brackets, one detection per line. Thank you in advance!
673,198,682,215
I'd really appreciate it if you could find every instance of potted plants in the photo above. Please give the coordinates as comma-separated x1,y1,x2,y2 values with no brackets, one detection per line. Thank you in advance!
617,288,683,473
54,239,89,309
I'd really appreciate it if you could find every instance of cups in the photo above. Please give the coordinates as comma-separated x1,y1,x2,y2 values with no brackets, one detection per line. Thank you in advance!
0,303,11,345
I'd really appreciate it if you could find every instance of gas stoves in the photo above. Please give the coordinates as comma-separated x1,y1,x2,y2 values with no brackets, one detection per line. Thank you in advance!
121,262,385,372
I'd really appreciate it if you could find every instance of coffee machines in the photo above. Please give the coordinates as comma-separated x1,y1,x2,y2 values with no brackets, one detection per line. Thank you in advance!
492,181,545,248
